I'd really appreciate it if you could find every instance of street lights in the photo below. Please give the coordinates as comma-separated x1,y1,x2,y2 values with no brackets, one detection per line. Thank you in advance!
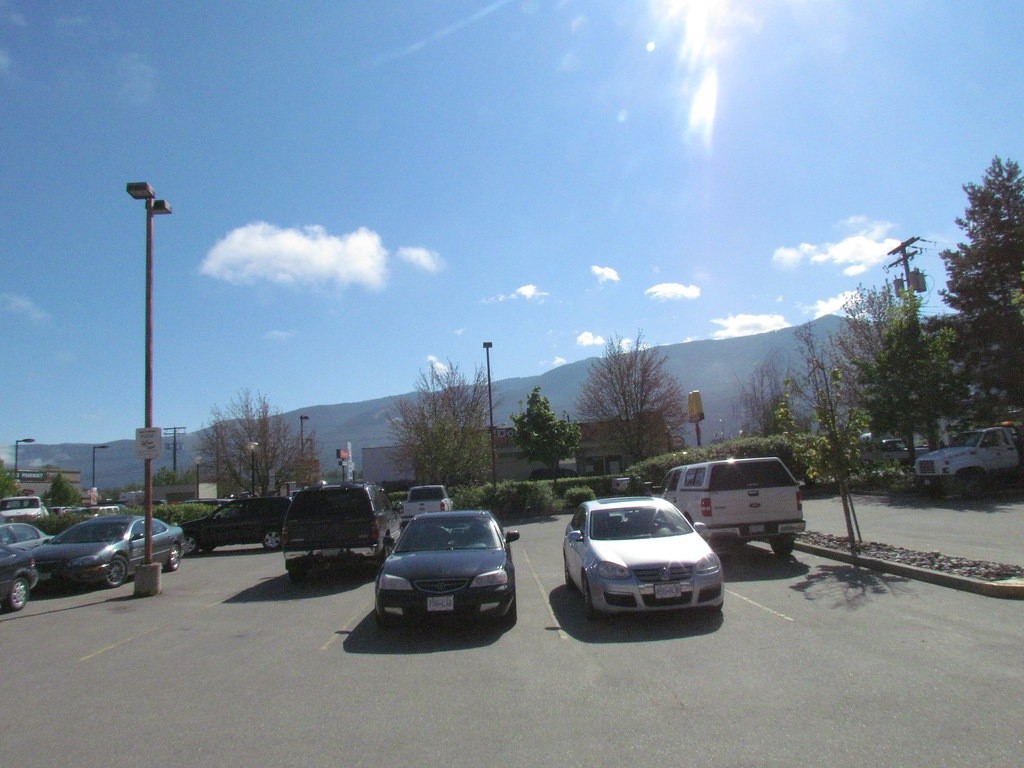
125,181,172,558
300,415,310,452
247,441,259,497
193,455,202,499
484,342,497,488
92,445,108,488
14,439,36,479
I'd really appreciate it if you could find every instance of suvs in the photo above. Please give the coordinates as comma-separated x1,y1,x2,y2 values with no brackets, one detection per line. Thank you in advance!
0,495,51,524
175,495,292,557
658,456,806,559
282,481,405,584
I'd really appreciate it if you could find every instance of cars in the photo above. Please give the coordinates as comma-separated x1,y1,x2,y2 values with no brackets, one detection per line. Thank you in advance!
879,438,914,466
375,509,521,629
0,540,40,612
48,503,129,516
26,513,187,589
563,496,724,620
0,522,56,553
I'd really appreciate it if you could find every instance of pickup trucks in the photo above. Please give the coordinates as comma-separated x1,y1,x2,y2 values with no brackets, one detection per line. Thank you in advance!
401,484,453,526
915,424,1024,503
